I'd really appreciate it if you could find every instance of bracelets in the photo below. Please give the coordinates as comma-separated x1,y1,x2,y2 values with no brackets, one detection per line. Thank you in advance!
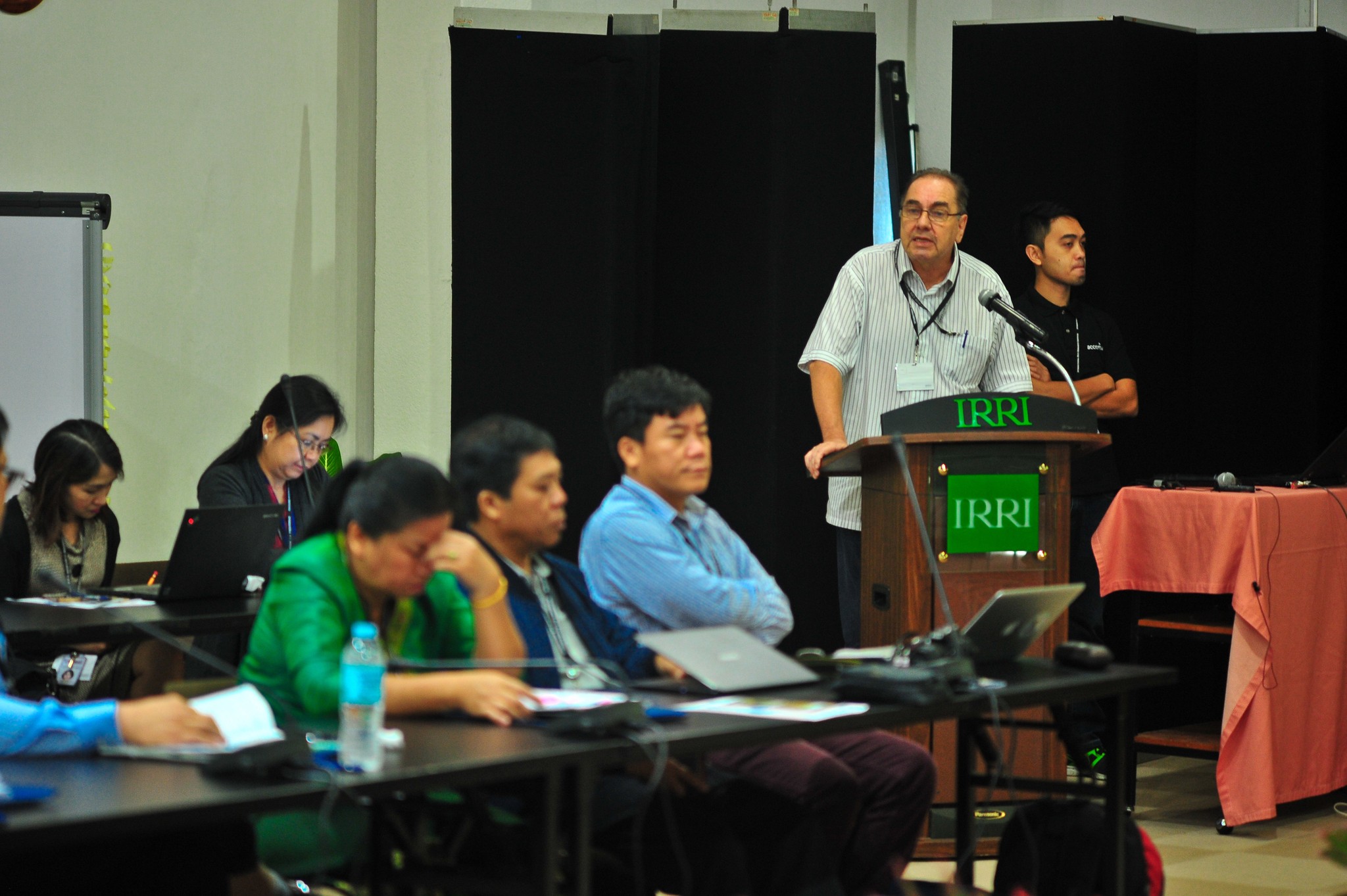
470,576,510,610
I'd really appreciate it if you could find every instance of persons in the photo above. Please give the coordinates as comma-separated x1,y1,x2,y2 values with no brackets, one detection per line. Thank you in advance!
796,167,1032,648
1011,201,1140,779
0,367,936,896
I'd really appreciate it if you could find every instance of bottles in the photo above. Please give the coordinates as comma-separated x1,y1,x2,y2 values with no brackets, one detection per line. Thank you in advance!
337,622,387,773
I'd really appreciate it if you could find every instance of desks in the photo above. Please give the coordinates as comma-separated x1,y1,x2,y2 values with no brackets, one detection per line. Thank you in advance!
0,645,1178,896
1086,476,1347,838
0,582,263,679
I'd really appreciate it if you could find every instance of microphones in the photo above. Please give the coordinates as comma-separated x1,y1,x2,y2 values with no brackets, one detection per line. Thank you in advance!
1154,472,1237,488
37,570,308,783
280,374,316,517
390,654,643,740
977,289,1049,343
836,434,974,705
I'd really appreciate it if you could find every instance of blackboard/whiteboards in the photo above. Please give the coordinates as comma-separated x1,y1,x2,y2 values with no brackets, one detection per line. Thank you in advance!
0,190,103,505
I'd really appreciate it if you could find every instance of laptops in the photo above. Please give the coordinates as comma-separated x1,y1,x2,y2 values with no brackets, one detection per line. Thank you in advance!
956,582,1085,660
618,626,822,696
89,502,286,603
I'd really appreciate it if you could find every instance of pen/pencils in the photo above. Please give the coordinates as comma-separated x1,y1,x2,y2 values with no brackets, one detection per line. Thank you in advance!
146,570,158,586
70,591,112,601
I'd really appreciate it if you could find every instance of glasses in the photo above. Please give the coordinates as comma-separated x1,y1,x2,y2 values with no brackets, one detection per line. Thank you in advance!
899,204,965,222
275,418,333,457
0,464,24,499
